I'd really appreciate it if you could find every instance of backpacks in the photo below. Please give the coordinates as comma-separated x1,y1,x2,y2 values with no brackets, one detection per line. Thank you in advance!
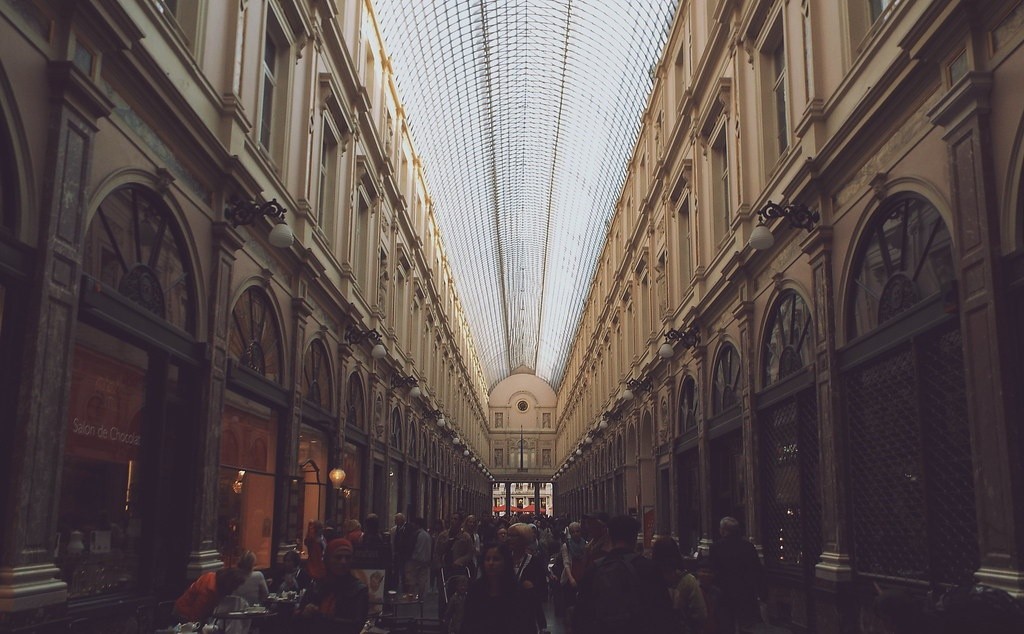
593,556,648,634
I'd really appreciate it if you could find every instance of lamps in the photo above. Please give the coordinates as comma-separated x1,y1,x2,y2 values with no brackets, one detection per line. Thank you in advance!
224,198,294,248
472,458,483,468
748,201,821,249
478,464,486,473
453,442,469,456
327,323,387,498
622,378,653,402
422,407,445,428
659,326,701,359
598,410,623,428
576,442,592,455
559,465,572,473
463,450,476,463
392,373,421,399
551,472,561,483
564,459,576,468
485,470,495,483
585,429,604,444
441,430,460,445
569,451,581,462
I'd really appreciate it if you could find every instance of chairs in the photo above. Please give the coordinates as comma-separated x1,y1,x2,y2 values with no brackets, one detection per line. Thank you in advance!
390,589,468,634
379,556,430,626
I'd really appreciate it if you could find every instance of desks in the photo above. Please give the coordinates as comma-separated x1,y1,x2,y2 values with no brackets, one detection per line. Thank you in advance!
264,595,303,630
213,610,280,634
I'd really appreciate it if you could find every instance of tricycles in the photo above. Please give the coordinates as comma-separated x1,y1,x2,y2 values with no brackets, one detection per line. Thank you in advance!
359,582,448,634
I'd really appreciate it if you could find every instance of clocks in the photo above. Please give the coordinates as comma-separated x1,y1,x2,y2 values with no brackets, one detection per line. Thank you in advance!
518,400,529,411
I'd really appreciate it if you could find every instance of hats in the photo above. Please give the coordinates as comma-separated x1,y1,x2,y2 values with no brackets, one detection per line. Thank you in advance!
326,539,354,564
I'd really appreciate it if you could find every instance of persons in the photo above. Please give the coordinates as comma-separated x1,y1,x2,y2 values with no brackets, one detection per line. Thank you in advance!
168,508,776,634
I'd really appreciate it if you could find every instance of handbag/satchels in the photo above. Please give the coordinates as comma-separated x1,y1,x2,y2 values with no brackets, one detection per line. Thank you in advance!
546,540,574,591
